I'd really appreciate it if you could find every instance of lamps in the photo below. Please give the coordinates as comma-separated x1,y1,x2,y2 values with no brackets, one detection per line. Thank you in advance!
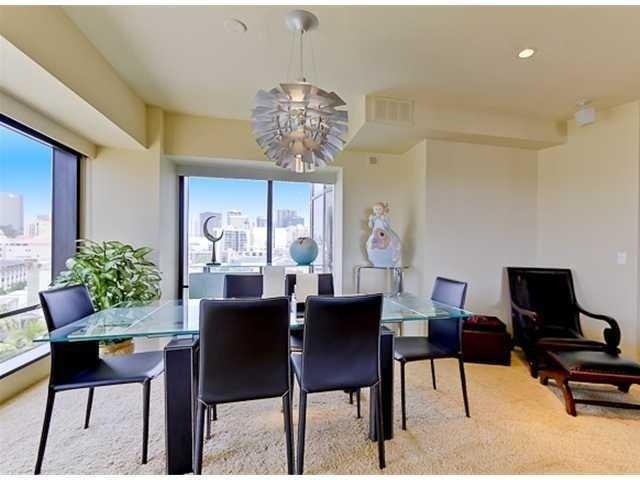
194,297,295,475
290,293,386,475
505,265,622,378
286,274,334,351
223,274,288,301
35,284,164,475
393,277,469,430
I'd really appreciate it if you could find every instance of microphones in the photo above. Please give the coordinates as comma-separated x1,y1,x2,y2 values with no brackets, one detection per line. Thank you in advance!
542,349,640,416
460,316,511,366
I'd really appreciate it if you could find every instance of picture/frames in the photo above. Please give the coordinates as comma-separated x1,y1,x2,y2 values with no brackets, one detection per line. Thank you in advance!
190,264,314,274
32,291,478,475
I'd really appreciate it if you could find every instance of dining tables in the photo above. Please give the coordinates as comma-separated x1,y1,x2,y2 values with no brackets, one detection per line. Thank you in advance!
49,238,163,355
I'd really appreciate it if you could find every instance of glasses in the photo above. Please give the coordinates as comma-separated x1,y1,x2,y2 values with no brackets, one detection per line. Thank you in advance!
251,9,349,175
574,98,596,125
